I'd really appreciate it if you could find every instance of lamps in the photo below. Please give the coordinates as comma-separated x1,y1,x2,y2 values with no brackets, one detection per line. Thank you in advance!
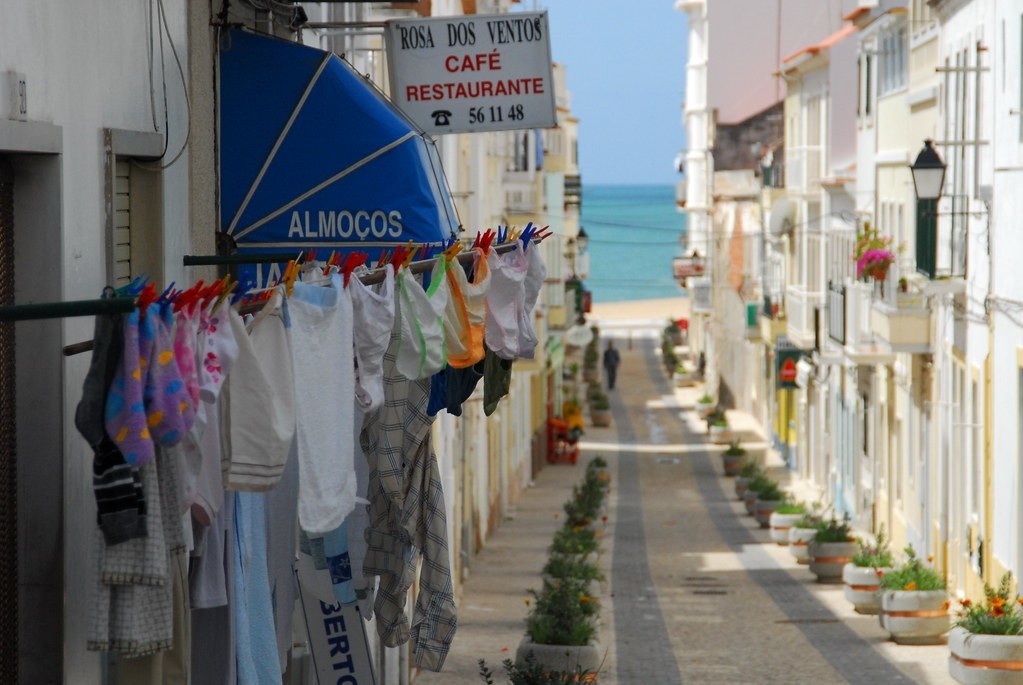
908,138,988,218
576,225,590,255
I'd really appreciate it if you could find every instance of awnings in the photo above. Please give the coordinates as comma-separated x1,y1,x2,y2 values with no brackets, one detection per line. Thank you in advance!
216,25,458,298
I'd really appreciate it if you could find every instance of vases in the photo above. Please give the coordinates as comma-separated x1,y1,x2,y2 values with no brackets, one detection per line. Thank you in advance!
842,562,896,616
789,526,817,564
946,617,1023,685
808,539,860,585
515,640,600,674
744,490,758,516
878,590,951,646
557,552,601,565
542,575,603,597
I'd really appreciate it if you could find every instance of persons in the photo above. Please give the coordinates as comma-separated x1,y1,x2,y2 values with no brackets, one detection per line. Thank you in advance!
604,340,621,389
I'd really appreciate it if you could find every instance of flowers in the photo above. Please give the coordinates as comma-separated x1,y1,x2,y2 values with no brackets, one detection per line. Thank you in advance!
748,468,770,491
523,468,613,647
477,644,611,685
940,570,1023,646
852,222,907,284
852,522,897,570
775,491,856,543
872,542,946,592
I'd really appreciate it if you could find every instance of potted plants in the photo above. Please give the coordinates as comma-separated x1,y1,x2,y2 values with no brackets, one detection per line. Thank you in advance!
769,512,807,547
581,317,728,445
753,484,786,530
735,455,761,500
723,447,749,478
